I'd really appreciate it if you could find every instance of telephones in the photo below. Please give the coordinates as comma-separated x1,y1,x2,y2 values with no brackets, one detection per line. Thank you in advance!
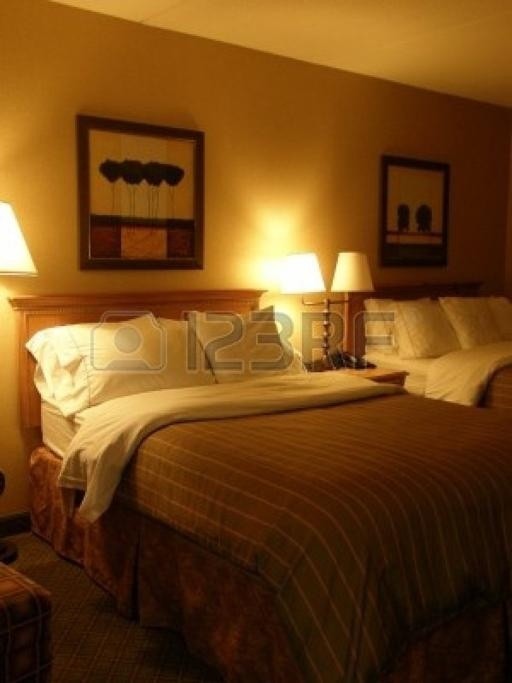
339,350,377,369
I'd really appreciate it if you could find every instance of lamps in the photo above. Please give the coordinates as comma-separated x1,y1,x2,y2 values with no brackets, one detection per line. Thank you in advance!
0,201,40,276
280,249,376,368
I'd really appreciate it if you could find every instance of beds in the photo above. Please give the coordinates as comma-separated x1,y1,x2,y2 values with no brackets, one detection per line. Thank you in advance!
344,276,512,408
12,290,512,682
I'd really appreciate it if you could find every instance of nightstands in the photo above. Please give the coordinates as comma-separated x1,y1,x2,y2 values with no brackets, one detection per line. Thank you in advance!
335,353,410,388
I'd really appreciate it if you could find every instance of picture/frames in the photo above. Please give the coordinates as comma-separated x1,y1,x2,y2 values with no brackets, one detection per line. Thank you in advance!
377,153,450,268
77,114,204,268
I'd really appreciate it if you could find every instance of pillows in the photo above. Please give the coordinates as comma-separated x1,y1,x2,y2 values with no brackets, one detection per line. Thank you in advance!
194,311,309,385
29,317,216,418
367,299,397,355
383,299,459,355
442,294,512,345
